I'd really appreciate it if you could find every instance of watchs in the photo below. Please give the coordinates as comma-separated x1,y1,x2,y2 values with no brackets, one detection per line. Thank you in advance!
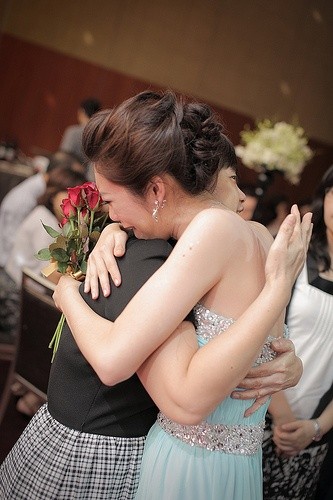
312,419,321,441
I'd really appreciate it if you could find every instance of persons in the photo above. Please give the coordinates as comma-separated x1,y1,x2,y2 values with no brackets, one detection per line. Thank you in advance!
0,98,323,416
0,131,313,500
52,90,289,500
263,167,333,498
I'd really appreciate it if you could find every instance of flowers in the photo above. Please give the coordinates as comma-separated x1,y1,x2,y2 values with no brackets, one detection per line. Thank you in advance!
235,113,315,186
33,182,109,362
